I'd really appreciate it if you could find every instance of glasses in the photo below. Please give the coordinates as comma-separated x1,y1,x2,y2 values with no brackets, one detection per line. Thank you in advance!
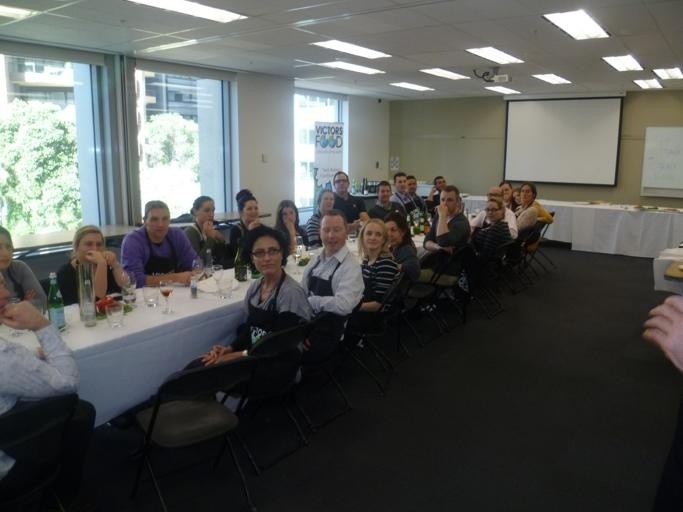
251,247,281,258
483,207,499,212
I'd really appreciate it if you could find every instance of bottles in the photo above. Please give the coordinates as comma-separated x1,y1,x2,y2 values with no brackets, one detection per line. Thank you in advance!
46,272,66,332
405,208,432,237
233,238,261,281
351,177,369,196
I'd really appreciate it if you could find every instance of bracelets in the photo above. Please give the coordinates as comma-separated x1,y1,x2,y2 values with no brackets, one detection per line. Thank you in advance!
241,350,248,358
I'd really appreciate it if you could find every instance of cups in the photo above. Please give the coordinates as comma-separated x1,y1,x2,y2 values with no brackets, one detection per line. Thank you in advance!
187,252,240,301
291,233,314,268
119,270,175,314
6,295,44,338
76,260,97,327
348,230,357,249
104,302,125,328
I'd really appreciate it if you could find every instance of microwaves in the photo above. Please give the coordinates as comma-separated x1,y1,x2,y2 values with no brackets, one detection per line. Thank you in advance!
364,181,382,196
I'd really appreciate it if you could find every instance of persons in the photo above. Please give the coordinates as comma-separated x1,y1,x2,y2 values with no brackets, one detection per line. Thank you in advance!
301,171,554,338
639,293,683,372
120,199,207,289
155,224,311,398
294,208,363,354
272,199,306,257
223,195,271,264
52,223,131,306
0,271,78,480
0,222,43,310
184,196,232,272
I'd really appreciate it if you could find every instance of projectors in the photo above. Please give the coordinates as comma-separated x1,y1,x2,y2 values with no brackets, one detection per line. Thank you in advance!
490,74,512,83
